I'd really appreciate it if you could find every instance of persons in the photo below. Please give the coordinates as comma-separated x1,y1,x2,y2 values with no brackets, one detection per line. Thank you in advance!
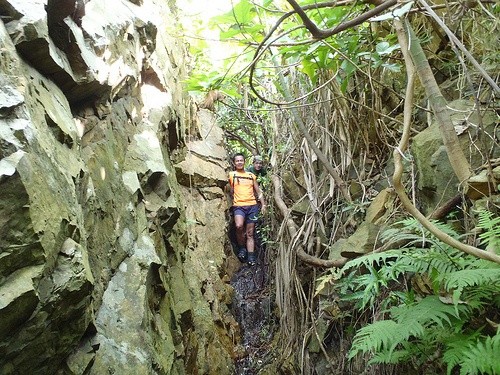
228,153,266,266
246,156,267,177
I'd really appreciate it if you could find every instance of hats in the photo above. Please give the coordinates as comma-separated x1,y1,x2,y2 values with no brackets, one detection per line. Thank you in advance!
253,155,263,164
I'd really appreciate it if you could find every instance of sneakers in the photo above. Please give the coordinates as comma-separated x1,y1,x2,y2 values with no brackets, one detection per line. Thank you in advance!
238,248,246,258
247,256,256,265
